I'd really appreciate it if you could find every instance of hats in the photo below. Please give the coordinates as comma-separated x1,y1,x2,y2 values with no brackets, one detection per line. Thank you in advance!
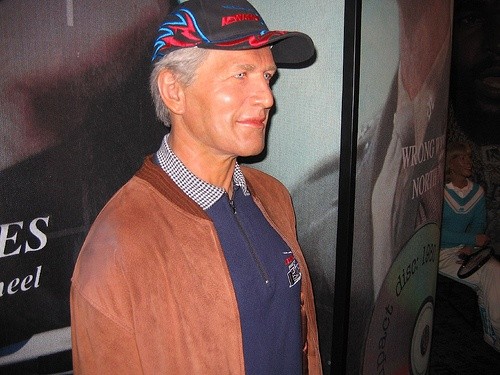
150,0,315,64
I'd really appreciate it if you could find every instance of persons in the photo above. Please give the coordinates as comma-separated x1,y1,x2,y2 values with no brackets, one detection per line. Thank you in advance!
346,0,452,342
68,0,327,375
437,141,500,351
0,0,184,375
